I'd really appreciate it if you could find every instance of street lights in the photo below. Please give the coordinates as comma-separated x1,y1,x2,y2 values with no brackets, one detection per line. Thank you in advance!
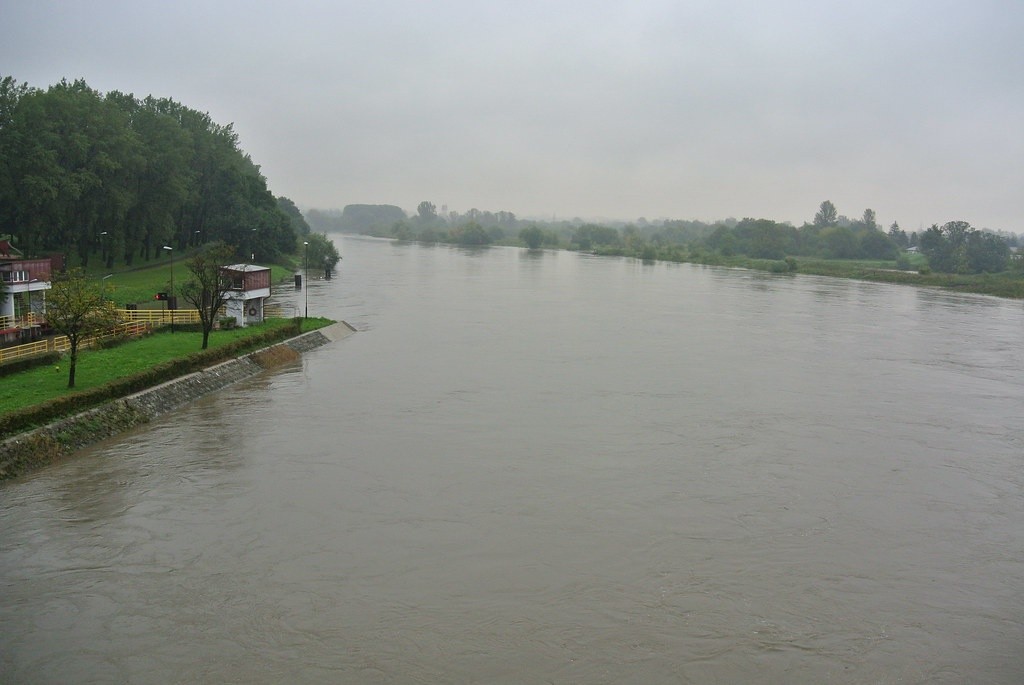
101,274,113,292
163,245,175,333
302,239,310,320
249,227,257,263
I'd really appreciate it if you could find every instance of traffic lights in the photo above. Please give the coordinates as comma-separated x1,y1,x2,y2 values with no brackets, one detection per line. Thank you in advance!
155,292,168,300
